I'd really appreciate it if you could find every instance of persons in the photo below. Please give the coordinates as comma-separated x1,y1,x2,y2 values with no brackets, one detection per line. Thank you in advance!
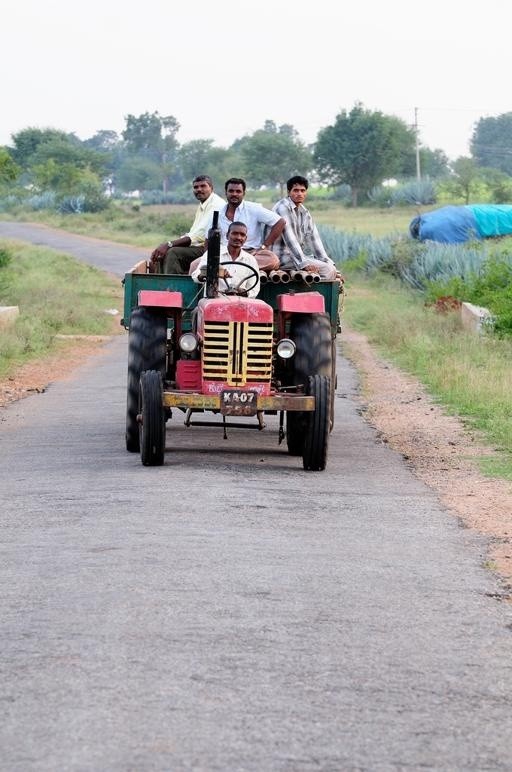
151,175,345,299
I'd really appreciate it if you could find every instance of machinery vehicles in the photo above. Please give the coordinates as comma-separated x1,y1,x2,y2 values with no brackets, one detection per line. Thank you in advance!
121,211,343,471
